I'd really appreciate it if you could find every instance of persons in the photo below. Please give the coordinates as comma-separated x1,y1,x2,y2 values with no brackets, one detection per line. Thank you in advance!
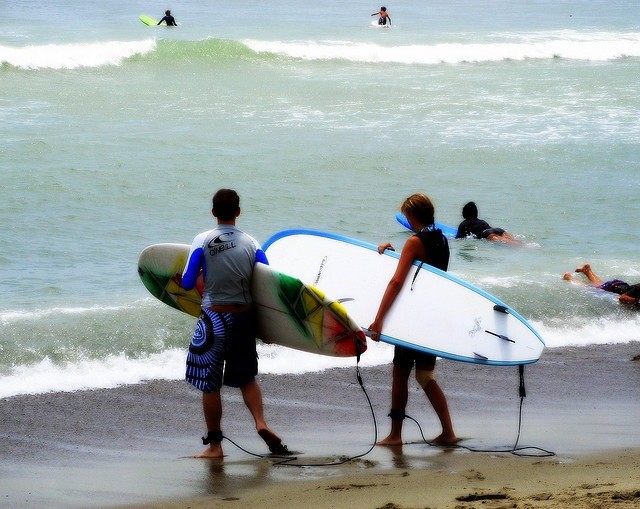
180,188,282,457
455,202,523,246
371,6,391,26
368,193,458,445
157,9,178,26
564,263,640,309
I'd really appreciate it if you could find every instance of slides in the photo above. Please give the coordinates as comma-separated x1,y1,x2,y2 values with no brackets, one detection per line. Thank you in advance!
372,20,389,28
262,230,545,365
396,213,458,238
140,15,166,26
138,244,367,357
